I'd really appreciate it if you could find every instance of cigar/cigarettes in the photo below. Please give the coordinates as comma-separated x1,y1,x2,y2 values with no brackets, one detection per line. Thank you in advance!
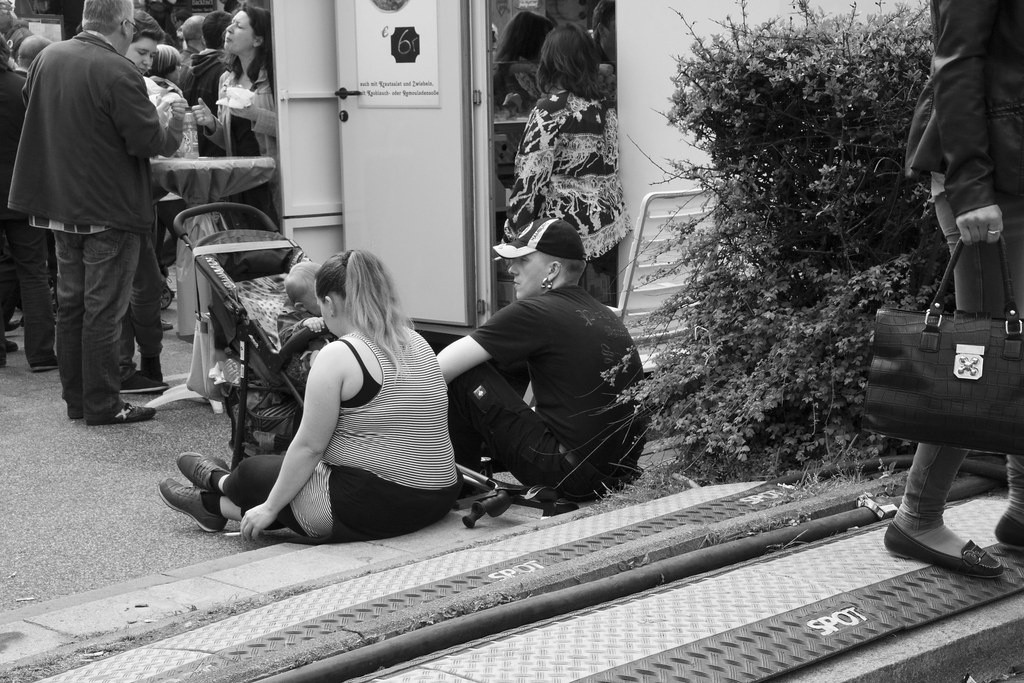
224,532,242,537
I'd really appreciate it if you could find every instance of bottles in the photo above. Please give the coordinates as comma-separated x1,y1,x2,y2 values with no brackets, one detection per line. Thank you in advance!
182,112,199,160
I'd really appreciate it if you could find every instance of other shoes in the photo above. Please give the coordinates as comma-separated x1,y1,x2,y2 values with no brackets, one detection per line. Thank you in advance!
0,341,18,365
30,359,59,371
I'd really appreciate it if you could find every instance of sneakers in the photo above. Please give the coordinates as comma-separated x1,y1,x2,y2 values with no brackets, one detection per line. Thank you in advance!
158,453,233,534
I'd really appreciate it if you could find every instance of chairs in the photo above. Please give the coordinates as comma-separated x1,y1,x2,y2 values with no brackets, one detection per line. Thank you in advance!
598,185,728,374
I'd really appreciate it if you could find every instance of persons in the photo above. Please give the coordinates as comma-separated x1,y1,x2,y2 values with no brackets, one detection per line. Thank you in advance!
190,6,282,234
119,7,185,393
589,0,617,72
0,0,272,98
0,34,60,371
435,217,648,505
545,0,589,32
187,10,232,158
158,247,458,544
275,258,336,392
489,10,554,110
7,0,163,425
884,0,1024,580
499,22,634,263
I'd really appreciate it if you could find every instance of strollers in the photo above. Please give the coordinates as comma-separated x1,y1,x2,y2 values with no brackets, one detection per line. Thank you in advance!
171,202,340,472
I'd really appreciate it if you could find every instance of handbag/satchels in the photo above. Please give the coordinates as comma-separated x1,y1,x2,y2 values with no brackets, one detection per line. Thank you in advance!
858,308,1024,457
186,311,226,402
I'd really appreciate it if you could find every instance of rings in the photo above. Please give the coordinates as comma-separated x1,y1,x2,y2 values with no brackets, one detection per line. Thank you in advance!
988,229,1000,235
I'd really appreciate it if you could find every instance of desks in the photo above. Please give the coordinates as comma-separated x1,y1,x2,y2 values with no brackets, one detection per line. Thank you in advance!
147,156,278,345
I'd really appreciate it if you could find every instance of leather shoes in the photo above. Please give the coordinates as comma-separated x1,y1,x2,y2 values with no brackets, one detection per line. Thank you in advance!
68,402,157,425
117,371,170,394
161,323,173,331
4,320,20,331
884,504,1024,579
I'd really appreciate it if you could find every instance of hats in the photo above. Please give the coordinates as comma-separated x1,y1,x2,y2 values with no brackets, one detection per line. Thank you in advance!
493,217,586,260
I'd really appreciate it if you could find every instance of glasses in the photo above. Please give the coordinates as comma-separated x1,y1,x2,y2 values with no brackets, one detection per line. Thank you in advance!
120,18,139,34
176,64,183,71
